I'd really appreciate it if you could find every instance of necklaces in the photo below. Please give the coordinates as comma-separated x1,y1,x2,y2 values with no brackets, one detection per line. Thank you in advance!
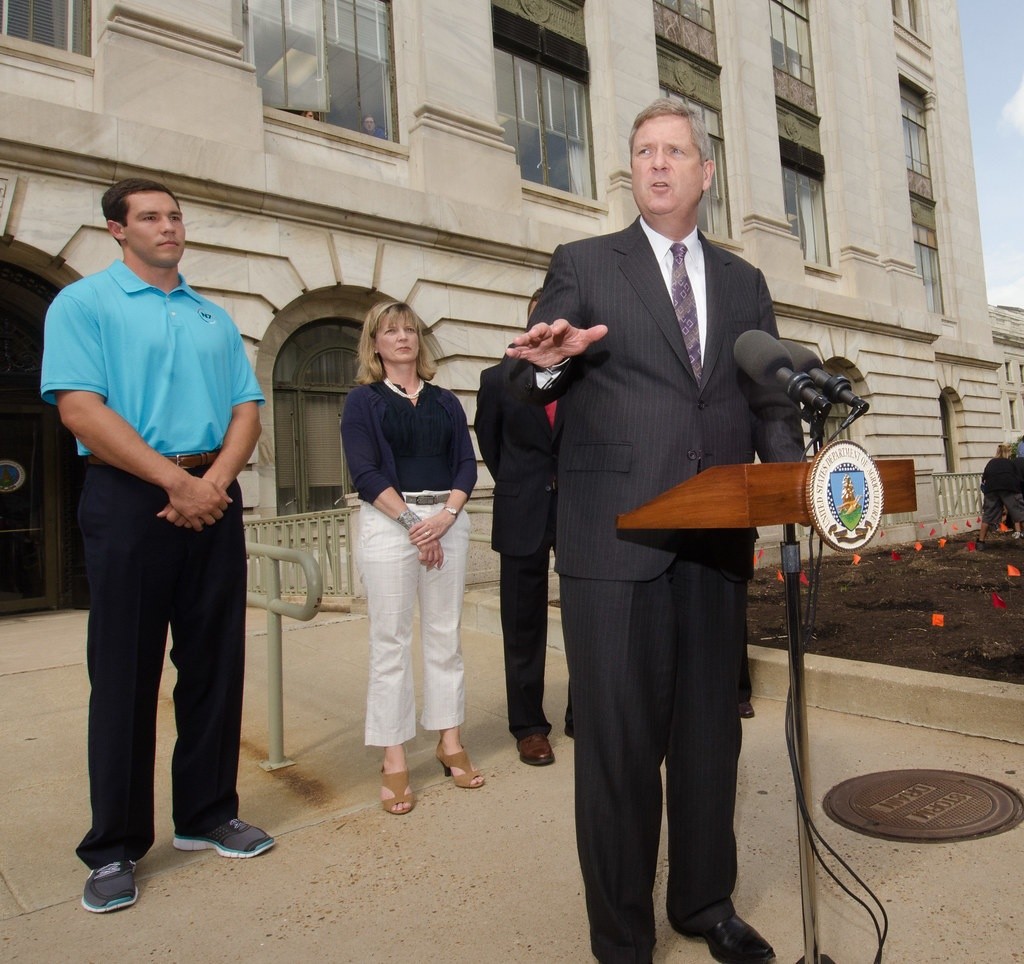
383,377,427,399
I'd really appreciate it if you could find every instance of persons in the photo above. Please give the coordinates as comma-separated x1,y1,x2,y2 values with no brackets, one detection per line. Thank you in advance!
40,180,277,912
474,285,575,767
976,434,1024,553
505,100,808,963
339,300,479,816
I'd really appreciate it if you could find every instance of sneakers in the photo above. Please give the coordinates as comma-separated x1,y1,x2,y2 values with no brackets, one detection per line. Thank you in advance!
81,858,138,912
172,819,275,858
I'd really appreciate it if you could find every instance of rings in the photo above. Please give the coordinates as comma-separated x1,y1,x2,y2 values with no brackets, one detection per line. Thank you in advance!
425,531,431,536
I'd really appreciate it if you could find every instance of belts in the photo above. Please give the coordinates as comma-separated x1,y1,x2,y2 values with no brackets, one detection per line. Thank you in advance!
88,451,218,471
405,493,450,505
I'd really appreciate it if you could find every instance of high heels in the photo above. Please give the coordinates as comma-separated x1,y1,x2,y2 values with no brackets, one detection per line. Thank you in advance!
380,763,415,814
435,738,483,788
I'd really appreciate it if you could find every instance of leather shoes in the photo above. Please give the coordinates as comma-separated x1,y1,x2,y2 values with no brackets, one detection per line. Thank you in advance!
516,734,555,766
739,701,755,719
695,912,775,964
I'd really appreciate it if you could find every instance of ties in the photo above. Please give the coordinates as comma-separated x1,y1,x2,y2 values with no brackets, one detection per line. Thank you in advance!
669,242,701,391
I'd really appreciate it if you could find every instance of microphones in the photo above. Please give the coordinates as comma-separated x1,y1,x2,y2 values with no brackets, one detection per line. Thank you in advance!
778,339,870,413
734,330,832,413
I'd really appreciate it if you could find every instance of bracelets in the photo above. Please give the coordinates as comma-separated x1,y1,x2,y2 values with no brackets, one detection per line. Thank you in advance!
397,511,421,530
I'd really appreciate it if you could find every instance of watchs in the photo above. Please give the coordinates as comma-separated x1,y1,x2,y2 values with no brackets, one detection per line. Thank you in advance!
444,506,458,518
